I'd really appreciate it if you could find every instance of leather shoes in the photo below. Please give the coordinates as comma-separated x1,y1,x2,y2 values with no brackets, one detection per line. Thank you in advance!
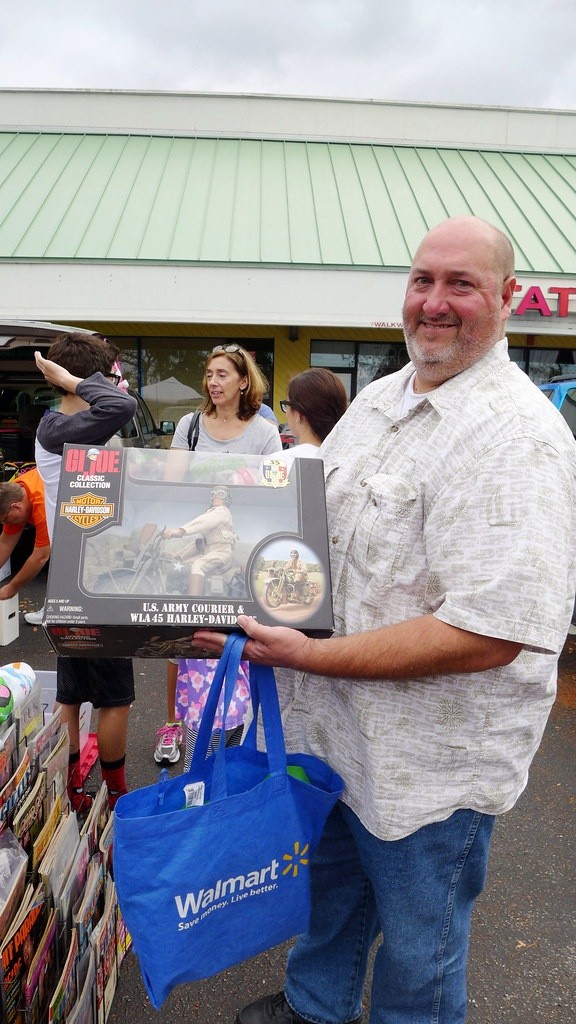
237,990,358,1024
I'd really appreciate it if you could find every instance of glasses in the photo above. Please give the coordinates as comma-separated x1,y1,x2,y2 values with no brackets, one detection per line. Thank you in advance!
280,400,296,413
289,555,297,557
103,372,120,386
210,490,227,500
213,345,239,354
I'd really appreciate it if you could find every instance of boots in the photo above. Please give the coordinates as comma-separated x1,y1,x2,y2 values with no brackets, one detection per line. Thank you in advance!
189,574,204,596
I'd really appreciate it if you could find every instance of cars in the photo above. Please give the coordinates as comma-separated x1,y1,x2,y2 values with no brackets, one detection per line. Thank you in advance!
538,374,575,436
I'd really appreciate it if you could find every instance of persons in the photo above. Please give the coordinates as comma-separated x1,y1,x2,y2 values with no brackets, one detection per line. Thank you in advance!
0,463,51,624
153,340,287,767
183,218,575,1023
270,358,350,461
36,332,139,824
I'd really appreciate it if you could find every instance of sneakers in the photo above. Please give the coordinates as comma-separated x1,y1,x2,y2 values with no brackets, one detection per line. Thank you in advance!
153,722,186,764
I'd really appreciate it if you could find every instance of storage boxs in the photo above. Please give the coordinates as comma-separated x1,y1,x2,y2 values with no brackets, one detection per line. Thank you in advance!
43,440,337,659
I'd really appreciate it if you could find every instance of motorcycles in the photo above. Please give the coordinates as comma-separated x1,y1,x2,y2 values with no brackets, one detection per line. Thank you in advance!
91,525,251,599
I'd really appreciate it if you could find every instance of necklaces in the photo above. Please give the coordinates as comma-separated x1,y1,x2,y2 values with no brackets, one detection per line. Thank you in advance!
214,412,237,423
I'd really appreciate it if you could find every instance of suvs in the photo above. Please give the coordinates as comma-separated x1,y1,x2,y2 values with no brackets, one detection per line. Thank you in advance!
0,320,176,481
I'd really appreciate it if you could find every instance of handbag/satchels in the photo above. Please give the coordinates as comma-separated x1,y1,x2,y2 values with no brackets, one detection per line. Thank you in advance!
113,635,346,1010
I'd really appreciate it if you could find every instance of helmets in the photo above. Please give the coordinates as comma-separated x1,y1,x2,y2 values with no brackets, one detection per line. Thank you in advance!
209,486,231,506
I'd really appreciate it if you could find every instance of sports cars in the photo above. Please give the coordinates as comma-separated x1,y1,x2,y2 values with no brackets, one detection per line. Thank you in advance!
264,566,315,608
